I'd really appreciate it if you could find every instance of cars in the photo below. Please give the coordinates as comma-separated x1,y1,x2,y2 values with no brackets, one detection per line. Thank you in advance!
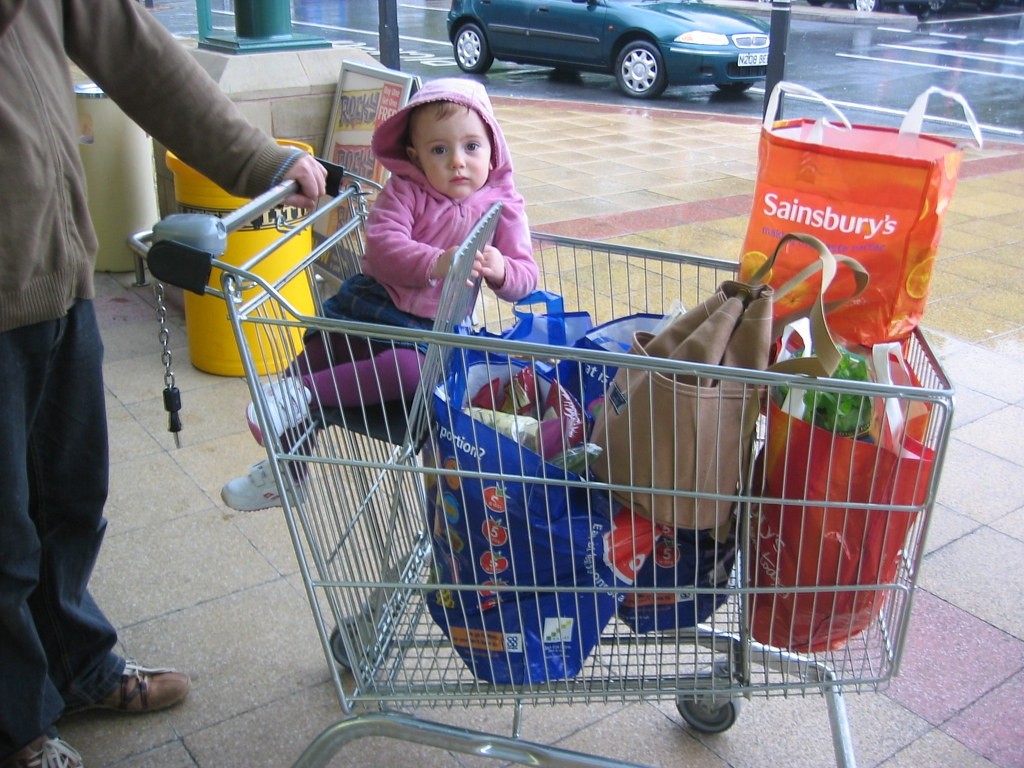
446,0,773,99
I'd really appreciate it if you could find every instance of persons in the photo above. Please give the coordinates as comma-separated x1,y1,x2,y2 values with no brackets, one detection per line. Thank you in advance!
221,77,540,512
1,0,327,768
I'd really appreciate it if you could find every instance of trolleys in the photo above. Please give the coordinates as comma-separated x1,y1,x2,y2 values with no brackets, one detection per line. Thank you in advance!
129,147,955,768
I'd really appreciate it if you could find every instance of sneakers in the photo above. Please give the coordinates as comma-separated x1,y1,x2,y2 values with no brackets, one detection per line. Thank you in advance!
245,375,312,448
60,659,190,716
0,733,85,768
221,457,307,512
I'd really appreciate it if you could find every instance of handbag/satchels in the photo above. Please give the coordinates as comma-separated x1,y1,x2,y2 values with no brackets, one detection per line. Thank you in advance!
738,77,983,347
444,289,594,403
426,327,663,685
576,313,741,632
591,233,870,529
741,317,937,652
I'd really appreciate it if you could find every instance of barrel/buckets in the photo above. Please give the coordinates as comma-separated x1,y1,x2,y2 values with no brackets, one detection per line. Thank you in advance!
165,138,316,377
